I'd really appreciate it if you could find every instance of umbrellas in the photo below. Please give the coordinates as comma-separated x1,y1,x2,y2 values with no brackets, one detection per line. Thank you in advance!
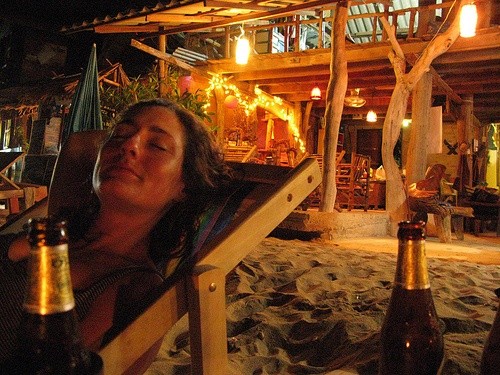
62,43,103,141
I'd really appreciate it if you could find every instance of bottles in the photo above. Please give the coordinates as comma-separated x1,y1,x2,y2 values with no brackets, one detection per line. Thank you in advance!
379,222,444,375
480,311,500,375
6,218,90,375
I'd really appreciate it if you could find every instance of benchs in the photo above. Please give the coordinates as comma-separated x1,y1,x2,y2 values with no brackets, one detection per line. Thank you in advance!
406,179,474,244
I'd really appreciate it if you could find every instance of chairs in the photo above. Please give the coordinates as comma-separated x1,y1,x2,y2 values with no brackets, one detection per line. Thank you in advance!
0,144,370,375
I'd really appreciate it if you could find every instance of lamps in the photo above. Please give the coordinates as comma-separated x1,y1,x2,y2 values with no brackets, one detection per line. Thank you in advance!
310,83,321,101
366,92,378,122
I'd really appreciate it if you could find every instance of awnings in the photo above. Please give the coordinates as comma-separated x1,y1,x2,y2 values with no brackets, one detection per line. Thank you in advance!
60,0,389,35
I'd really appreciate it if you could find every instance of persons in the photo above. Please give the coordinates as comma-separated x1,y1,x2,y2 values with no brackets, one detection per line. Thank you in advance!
0,98,230,374
